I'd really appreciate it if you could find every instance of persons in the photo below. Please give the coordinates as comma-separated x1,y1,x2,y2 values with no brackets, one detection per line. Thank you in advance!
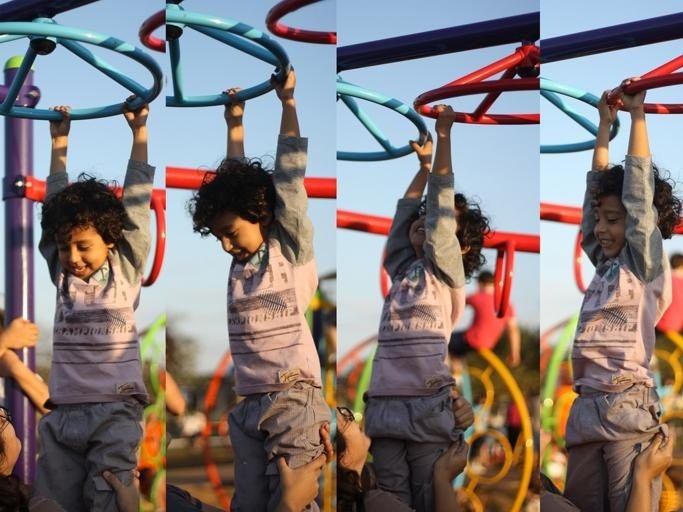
31,92,155,512
561,76,669,512
359,103,495,512
189,65,332,512
1,321,683,510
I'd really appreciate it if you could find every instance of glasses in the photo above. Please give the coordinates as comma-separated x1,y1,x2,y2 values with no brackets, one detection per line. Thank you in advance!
337,407,355,437
0,405,16,431
